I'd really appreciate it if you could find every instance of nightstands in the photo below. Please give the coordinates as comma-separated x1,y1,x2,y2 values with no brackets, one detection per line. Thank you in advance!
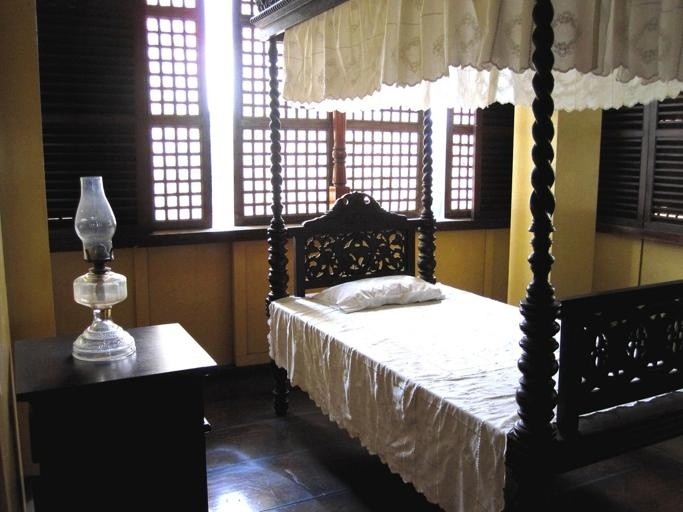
12,323,218,512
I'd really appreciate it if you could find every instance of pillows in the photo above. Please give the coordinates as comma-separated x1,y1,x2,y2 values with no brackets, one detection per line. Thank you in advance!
310,274,445,314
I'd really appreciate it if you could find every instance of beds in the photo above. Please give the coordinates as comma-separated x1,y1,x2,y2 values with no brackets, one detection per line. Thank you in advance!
250,0,683,512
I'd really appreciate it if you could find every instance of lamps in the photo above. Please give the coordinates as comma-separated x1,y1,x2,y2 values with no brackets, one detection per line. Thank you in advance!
71,177,136,361
